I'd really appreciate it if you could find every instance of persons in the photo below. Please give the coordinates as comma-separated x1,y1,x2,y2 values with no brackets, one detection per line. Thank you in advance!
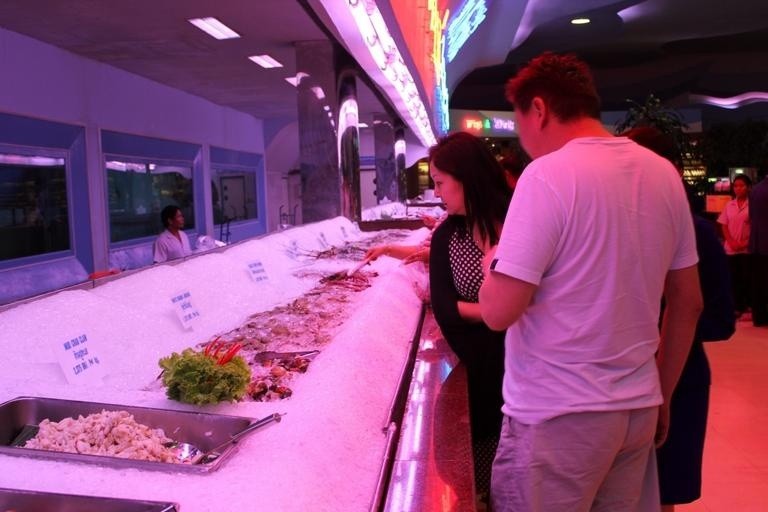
620,124,734,512
747,171,768,328
715,174,750,320
151,204,192,263
426,128,517,511
477,49,704,510
499,151,533,190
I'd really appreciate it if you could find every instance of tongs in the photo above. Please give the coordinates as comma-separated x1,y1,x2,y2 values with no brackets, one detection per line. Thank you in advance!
346,256,374,278
250,349,322,372
